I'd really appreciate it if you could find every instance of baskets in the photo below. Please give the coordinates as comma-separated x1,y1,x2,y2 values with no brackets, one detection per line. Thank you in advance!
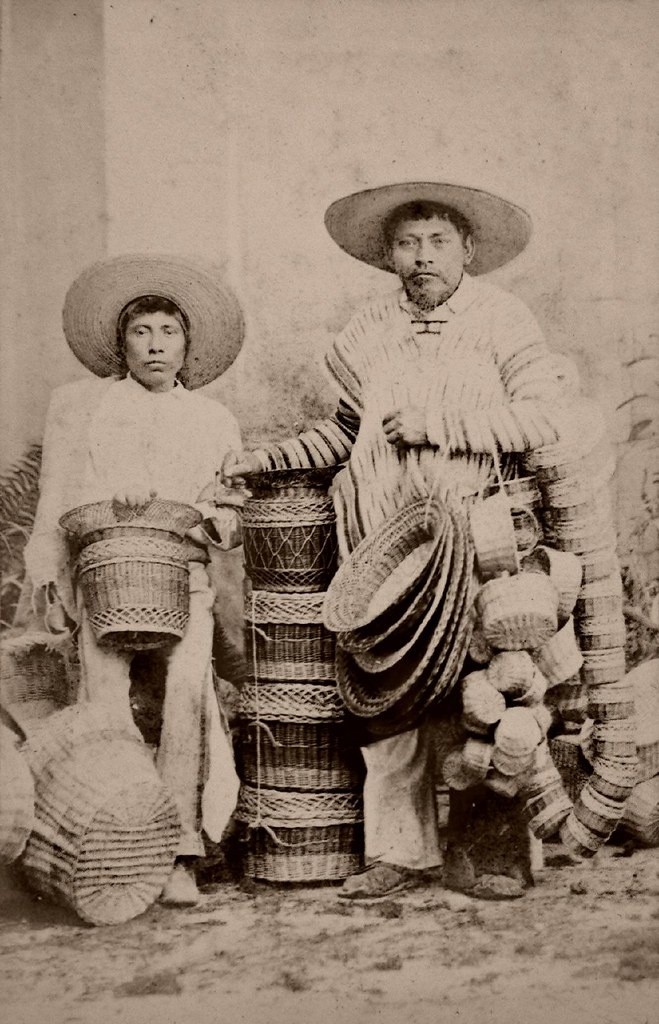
0,632,183,929
58,498,204,652
232,465,637,883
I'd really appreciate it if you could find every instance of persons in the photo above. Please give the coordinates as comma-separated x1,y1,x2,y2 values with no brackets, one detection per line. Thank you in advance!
219,200,564,898
24,294,252,907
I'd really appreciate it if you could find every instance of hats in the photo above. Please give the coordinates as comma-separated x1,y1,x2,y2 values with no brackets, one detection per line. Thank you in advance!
324,181,533,275
62,252,246,391
